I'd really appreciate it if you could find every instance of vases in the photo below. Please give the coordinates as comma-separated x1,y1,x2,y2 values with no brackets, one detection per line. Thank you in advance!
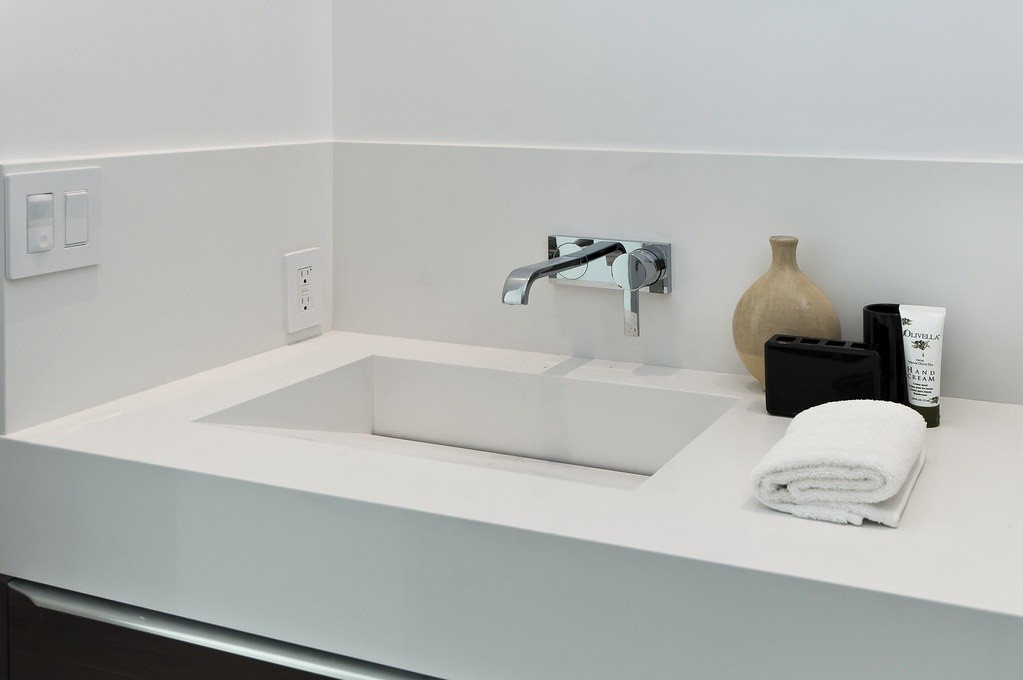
730,234,842,394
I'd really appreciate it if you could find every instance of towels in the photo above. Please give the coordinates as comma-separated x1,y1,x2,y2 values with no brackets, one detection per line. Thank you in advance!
748,397,930,530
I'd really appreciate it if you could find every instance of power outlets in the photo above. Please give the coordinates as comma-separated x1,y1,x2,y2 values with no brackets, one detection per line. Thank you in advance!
762,332,879,420
283,247,323,333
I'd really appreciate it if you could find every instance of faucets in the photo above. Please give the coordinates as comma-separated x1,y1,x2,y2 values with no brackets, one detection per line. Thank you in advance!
502,240,627,306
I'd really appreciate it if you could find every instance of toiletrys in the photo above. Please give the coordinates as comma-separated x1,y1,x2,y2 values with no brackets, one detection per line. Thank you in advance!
895,302,948,428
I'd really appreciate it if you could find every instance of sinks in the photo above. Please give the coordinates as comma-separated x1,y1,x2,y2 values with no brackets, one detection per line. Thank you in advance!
182,349,742,495
